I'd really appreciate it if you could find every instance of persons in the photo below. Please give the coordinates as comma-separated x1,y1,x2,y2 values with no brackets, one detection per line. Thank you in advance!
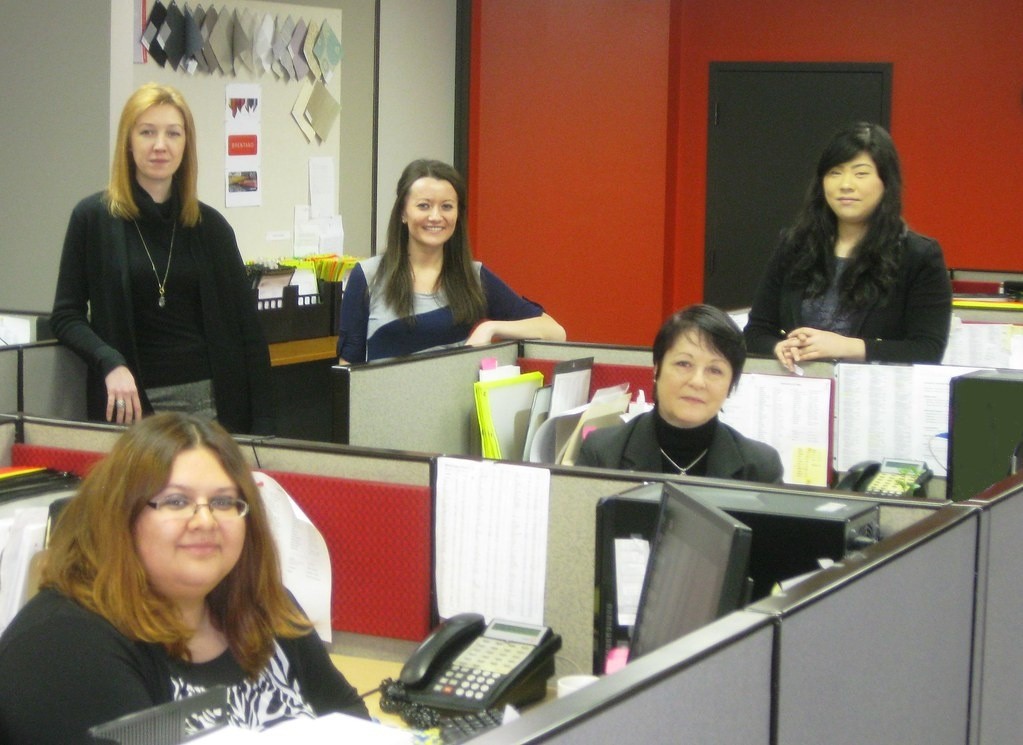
0,414,372,745
51,84,272,436
744,122,952,371
576,304,784,483
338,159,567,365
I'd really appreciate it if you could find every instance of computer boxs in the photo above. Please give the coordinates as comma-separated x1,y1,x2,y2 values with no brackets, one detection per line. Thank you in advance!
946,369,1023,502
591,480,881,678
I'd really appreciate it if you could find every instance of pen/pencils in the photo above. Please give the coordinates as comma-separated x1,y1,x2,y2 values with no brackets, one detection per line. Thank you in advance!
780,329,787,337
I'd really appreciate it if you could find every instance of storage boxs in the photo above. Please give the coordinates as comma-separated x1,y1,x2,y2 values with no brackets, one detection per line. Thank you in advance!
254,286,292,343
317,279,343,337
288,278,334,341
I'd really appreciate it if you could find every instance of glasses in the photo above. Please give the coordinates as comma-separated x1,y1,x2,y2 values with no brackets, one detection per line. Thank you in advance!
149,494,251,521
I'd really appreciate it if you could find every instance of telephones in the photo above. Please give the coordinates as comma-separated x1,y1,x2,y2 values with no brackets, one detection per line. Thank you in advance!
832,457,933,498
386,612,563,716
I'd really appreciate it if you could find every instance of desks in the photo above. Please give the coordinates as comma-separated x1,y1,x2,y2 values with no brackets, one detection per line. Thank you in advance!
0,293,1023,745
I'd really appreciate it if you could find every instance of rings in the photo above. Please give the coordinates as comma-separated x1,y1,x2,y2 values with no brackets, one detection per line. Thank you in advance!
116,400,126,408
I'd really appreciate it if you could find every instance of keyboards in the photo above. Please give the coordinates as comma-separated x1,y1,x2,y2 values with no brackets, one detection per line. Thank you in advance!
439,709,506,745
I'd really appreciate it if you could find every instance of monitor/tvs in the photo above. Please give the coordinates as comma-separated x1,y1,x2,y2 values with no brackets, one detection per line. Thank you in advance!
626,480,755,668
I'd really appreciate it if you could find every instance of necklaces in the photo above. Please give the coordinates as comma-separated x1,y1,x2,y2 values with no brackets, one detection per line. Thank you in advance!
660,448,708,476
134,219,176,307
